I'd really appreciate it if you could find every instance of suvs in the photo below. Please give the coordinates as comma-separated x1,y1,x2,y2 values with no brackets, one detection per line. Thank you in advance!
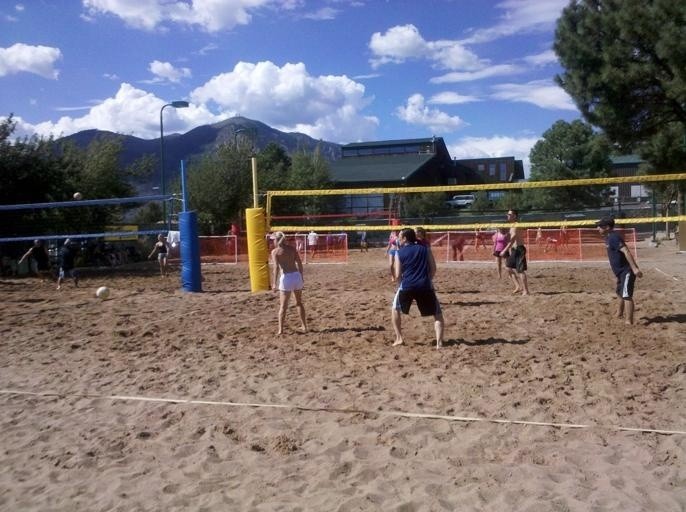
446,194,477,208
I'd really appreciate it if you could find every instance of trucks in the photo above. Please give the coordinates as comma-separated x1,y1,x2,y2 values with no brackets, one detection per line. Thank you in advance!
49,222,173,267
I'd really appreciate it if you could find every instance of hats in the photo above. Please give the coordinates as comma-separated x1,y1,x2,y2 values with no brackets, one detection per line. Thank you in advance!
594,216,616,226
268,232,283,240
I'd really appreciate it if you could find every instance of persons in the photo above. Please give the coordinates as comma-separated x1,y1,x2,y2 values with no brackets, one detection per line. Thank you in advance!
390,229,443,350
336,230,346,250
492,228,510,279
498,209,528,297
269,232,307,335
146,234,168,279
559,219,568,246
57,239,79,290
325,232,332,253
473,235,486,252
451,237,465,261
593,216,643,325
16,240,56,282
534,224,542,249
0,254,14,277
306,231,319,258
544,235,560,253
293,231,302,252
357,231,367,253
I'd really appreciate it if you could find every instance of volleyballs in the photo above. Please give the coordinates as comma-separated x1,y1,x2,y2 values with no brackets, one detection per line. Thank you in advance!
73,191,82,199
97,285,110,299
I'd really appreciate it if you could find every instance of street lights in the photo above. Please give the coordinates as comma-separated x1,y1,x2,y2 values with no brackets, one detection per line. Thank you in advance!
159,100,190,229
